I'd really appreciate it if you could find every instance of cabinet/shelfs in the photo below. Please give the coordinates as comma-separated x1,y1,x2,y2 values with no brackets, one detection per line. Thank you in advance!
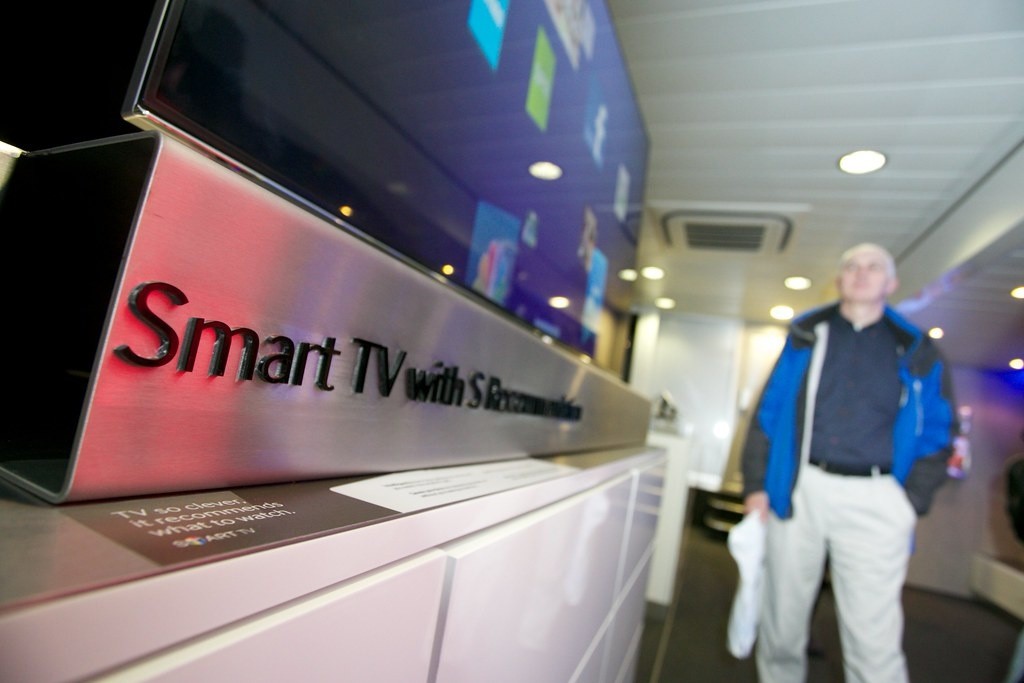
1,444,670,682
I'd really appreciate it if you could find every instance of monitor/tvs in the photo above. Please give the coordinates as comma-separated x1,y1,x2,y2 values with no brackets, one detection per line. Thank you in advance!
0,0,656,360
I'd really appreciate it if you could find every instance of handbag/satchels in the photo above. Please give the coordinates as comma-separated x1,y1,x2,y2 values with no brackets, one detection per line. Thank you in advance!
728,509,763,659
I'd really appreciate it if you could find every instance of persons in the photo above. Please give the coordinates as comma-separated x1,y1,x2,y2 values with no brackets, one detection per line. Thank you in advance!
739,243,957,683
506,207,597,348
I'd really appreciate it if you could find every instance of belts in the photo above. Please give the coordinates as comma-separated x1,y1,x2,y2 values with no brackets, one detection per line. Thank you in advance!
809,458,893,476
